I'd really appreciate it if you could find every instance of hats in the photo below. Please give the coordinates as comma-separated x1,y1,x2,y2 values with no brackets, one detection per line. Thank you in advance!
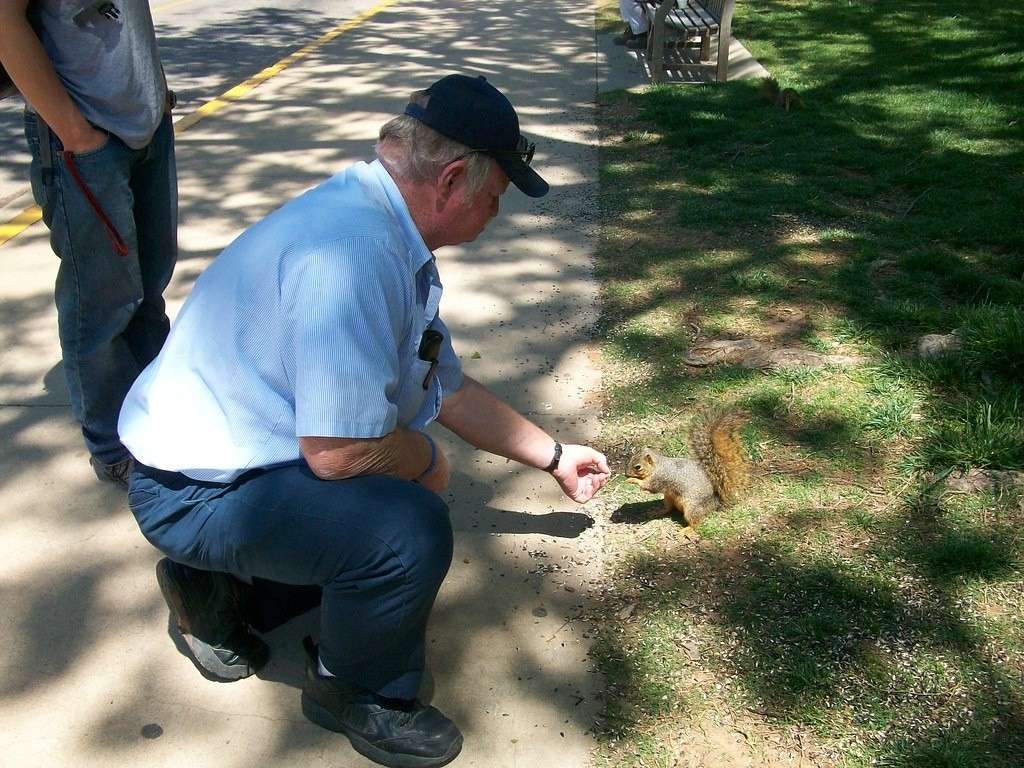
404,74,550,197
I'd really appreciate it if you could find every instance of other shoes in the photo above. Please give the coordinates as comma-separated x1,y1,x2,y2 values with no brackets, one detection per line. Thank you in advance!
90,457,132,488
626,34,647,48
614,28,633,45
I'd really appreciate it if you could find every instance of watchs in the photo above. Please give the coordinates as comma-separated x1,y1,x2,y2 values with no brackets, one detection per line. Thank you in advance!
166,90,177,109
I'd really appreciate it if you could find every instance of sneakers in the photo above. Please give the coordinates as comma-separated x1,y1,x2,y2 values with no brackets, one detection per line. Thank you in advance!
156,557,270,679
301,635,464,768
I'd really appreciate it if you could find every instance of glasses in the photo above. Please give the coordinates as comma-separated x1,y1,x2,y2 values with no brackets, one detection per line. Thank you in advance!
442,134,536,172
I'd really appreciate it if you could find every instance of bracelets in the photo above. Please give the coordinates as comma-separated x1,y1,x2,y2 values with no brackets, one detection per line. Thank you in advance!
542,440,562,472
412,431,436,481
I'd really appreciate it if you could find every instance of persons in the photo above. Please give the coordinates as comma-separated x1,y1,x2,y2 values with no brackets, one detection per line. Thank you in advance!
117,76,610,767
613,0,687,49
0,0,178,488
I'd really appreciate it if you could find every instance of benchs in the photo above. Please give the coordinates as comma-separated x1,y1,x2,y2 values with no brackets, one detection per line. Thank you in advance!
641,0,735,84
